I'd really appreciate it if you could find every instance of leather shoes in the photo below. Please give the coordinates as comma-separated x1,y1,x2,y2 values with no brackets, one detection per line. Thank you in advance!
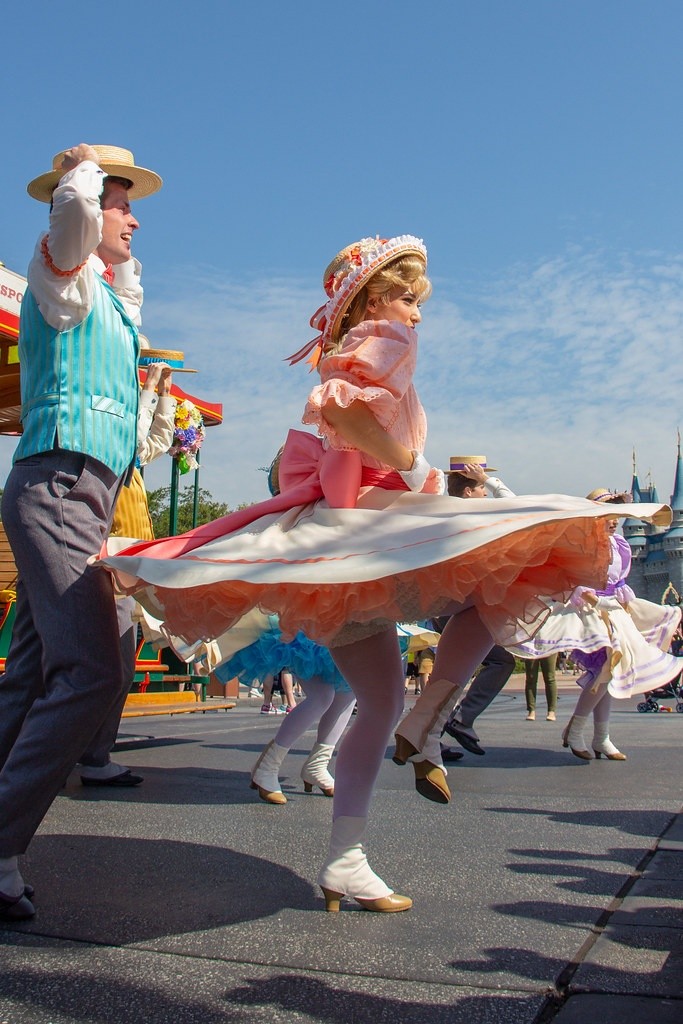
81,770,144,786
0,885,37,918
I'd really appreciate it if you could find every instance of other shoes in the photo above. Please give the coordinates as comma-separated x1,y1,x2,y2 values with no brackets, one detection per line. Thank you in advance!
445,716,485,755
526,712,535,720
439,740,463,762
546,711,556,721
404,688,421,695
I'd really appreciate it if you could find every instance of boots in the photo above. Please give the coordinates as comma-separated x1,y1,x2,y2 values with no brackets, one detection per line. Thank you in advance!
592,720,627,760
561,713,592,760
249,738,290,804
318,814,412,912
300,743,335,797
392,675,464,804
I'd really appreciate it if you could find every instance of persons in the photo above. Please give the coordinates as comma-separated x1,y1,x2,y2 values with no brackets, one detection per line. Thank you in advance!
433,456,517,760
506,489,683,760
525,652,557,721
558,652,579,675
143,606,443,803
1,142,161,919
90,235,671,914
77,363,174,789
404,617,439,694
250,666,302,715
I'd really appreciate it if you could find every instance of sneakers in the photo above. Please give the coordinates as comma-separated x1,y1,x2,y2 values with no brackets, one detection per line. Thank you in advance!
249,687,303,715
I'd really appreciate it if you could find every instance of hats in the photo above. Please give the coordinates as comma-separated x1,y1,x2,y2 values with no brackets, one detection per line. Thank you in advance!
443,455,498,474
267,438,325,497
283,235,427,375
26,145,164,204
586,488,633,504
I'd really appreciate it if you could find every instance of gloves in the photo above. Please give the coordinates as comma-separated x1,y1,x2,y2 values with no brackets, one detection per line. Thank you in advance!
594,595,622,611
396,448,446,495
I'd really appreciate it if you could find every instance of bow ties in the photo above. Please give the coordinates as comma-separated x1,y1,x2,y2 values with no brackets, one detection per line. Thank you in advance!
135,455,140,468
103,262,115,286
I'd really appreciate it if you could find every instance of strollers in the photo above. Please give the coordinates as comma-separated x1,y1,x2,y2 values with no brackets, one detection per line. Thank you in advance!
636,627,683,714
